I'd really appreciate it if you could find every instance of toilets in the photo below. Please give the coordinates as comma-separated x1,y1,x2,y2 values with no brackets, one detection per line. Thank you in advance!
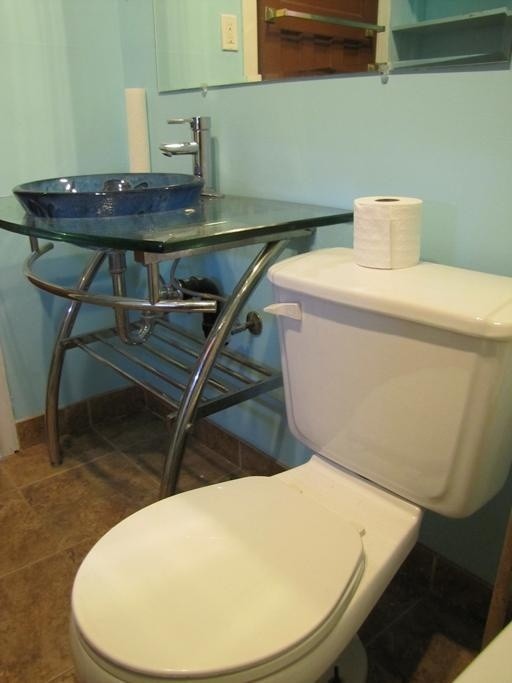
69,246,512,683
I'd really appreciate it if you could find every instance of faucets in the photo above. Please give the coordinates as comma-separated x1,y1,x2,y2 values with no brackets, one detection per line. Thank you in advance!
158,115,213,195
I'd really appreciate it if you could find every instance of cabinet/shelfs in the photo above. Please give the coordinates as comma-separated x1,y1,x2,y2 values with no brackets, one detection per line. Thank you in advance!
389,6,512,64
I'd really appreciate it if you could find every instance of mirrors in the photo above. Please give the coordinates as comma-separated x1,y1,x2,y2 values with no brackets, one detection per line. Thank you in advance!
152,0,512,94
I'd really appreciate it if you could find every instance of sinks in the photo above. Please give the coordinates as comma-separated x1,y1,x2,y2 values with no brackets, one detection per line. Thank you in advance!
11,174,201,250
